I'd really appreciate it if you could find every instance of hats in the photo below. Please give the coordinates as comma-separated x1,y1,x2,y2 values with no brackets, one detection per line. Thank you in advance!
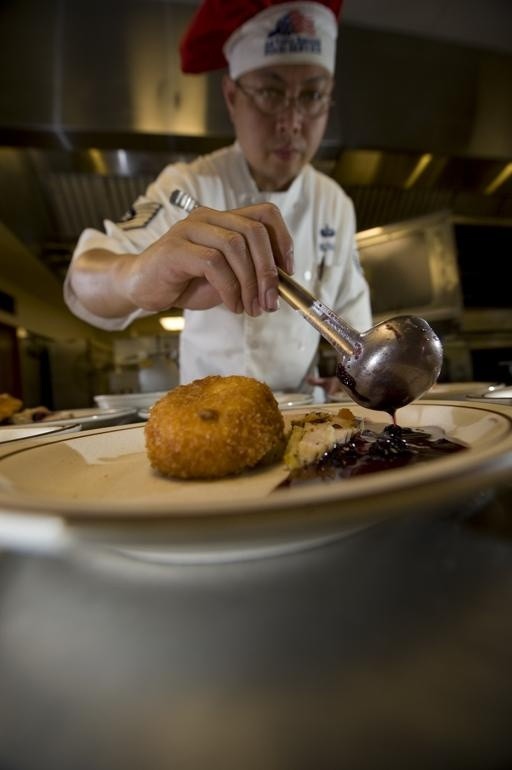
179,0,344,82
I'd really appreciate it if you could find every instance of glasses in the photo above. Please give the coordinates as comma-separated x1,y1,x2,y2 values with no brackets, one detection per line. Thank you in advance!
234,81,341,120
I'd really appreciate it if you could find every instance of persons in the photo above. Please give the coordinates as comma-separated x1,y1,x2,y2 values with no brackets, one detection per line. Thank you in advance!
61,59,377,404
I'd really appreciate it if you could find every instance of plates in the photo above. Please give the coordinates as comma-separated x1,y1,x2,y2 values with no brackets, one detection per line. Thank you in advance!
1,381,511,565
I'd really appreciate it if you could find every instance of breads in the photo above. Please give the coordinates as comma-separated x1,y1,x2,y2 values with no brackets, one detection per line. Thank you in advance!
143,374,284,479
